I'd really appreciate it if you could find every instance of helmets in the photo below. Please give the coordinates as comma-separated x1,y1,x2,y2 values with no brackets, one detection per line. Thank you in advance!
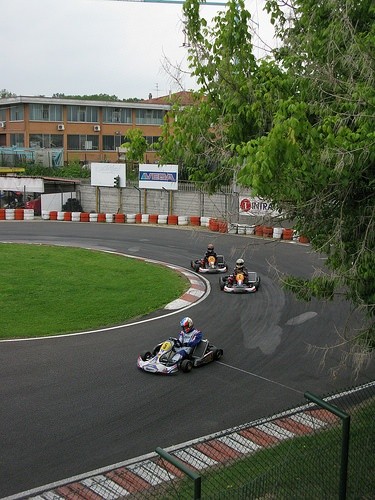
207,244,214,252
180,317,194,333
236,259,245,268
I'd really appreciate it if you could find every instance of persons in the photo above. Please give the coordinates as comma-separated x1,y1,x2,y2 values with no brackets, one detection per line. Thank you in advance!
227,258,248,288
171,317,202,365
203,243,218,268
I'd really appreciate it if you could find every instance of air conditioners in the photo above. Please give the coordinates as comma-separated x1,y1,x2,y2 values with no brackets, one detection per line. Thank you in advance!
93,125,100,131
0,121,5,128
57,124,64,131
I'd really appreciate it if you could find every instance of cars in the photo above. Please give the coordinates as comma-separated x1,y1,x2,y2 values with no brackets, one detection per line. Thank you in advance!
25,196,41,215
